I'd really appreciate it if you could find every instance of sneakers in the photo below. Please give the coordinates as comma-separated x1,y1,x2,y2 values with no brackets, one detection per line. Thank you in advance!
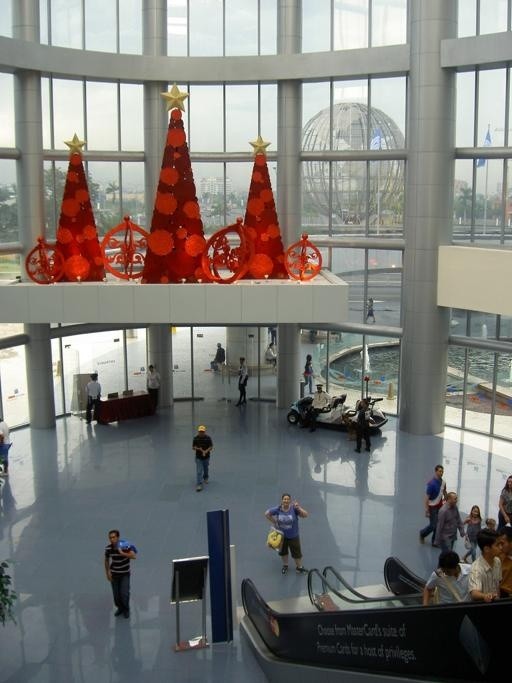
115,608,121,616
123,610,129,618
196,483,203,491
204,479,209,485
282,565,288,576
296,566,308,575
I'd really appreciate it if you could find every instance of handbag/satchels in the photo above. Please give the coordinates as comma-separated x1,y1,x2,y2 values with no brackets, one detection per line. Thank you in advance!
267,527,285,552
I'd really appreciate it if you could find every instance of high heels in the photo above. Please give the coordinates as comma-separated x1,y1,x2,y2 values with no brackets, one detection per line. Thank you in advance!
236,402,241,407
241,400,246,405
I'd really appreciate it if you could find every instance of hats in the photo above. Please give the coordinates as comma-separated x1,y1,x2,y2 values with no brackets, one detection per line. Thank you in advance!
437,551,459,569
198,426,206,431
316,385,323,387
217,343,221,347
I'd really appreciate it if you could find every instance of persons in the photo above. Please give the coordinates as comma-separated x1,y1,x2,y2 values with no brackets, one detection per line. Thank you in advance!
104,530,137,619
299,384,332,433
210,343,225,372
463,505,482,564
192,425,213,492
331,331,342,343
235,357,248,407
85,373,101,424
265,493,309,575
433,492,466,553
302,354,314,394
146,365,161,412
367,298,376,323
500,527,512,597
265,342,276,366
309,330,318,345
267,328,276,345
496,475,512,532
354,399,371,454
423,549,472,606
418,464,448,548
467,528,503,603
486,518,496,532
0,418,11,477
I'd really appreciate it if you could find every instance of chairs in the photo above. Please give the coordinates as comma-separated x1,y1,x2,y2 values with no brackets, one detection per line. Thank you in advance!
317,395,342,423
340,392,347,419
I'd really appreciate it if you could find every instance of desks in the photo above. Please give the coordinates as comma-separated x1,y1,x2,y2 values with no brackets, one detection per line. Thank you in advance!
96,389,151,423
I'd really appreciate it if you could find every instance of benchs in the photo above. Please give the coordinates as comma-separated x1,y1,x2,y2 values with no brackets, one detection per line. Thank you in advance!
216,361,274,376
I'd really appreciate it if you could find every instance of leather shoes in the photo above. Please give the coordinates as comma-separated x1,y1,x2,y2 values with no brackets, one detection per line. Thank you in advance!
300,425,308,428
309,428,316,432
354,449,360,452
365,448,370,451
